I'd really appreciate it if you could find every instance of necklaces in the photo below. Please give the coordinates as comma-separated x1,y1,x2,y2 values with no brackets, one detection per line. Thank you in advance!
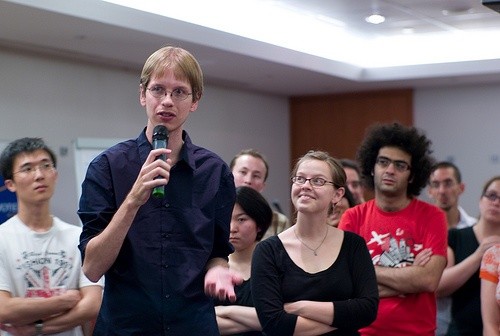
293,225,328,256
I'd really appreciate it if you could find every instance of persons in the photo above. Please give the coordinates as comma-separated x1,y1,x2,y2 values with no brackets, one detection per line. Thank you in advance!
480,242,500,336
251,150,379,336
427,162,478,336
337,122,448,336
0,137,105,336
327,187,354,227
338,158,364,205
213,186,273,336
436,176,500,336
78,46,242,336
230,151,290,243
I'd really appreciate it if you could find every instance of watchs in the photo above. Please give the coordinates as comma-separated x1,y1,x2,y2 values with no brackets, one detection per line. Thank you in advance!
34,319,45,335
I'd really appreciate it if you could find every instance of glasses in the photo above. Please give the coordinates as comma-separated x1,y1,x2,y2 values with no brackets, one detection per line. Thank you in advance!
9,160,56,176
290,176,341,190
375,156,411,172
482,190,499,202
142,83,193,102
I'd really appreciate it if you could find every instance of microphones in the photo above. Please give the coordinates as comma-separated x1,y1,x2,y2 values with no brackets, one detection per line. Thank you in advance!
152,124,169,199
